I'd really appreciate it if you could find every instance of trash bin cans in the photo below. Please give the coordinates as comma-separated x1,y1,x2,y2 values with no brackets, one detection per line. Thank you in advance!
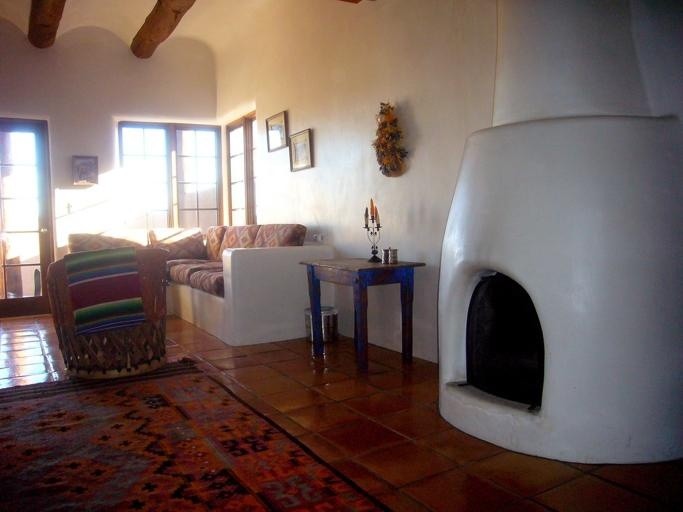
304,305,338,343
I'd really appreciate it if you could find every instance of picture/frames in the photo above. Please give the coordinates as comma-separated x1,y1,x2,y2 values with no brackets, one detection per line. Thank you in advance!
70,155,98,187
264,110,314,172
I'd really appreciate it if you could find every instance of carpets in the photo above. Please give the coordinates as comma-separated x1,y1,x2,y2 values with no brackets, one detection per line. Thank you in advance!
0,357,393,512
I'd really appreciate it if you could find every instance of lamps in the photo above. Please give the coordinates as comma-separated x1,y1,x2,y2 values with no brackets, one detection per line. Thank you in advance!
361,197,382,263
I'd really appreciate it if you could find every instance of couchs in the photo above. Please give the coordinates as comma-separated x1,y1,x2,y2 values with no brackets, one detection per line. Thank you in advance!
160,224,334,347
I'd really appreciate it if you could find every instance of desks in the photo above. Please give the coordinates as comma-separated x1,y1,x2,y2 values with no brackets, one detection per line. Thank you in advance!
298,258,426,371
